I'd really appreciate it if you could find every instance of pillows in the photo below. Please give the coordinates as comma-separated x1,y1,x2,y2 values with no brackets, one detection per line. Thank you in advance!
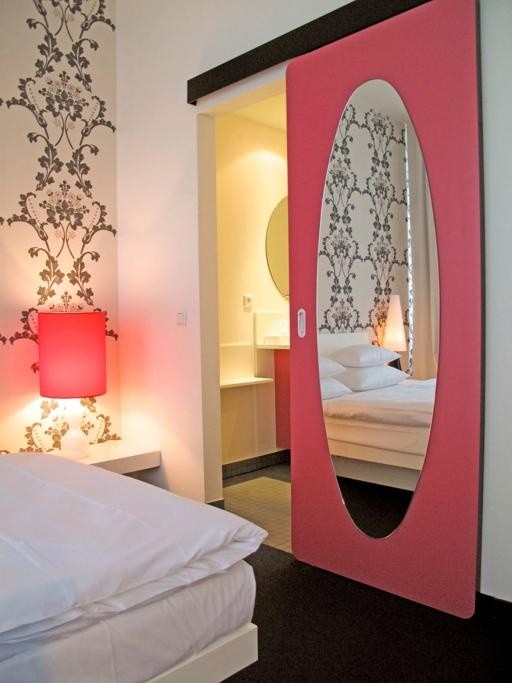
318,343,411,400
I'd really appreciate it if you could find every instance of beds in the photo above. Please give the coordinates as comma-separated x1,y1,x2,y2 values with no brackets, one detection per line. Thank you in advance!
1,450,261,681
318,332,436,492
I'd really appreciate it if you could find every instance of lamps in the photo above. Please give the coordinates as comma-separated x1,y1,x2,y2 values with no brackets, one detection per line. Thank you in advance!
381,293,407,369
37,307,109,401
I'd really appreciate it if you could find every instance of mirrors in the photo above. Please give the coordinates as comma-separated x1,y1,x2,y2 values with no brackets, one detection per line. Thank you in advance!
312,72,443,544
